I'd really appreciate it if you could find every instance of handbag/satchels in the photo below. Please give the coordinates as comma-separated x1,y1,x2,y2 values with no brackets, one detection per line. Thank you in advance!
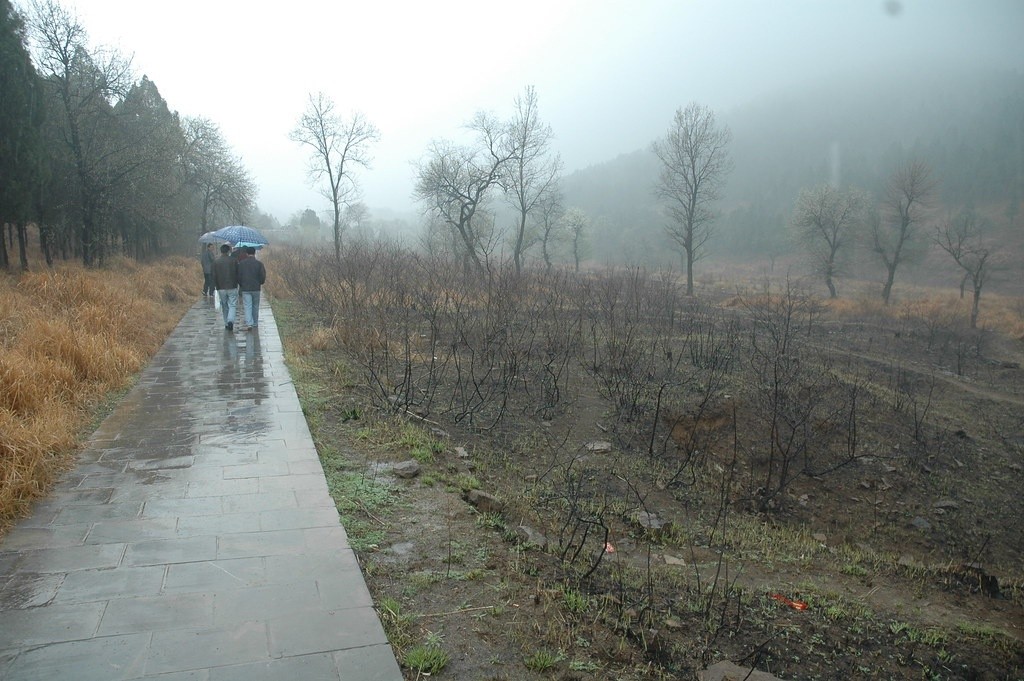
214,286,220,314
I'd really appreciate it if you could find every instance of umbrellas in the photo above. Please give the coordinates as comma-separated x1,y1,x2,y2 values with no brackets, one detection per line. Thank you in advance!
199,231,226,243
213,221,269,247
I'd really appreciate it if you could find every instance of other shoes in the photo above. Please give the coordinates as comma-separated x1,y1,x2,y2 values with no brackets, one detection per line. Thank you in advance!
202,292,207,297
210,294,215,297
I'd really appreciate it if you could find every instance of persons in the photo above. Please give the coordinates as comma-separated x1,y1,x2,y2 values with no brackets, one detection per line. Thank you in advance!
201,243,215,296
211,245,266,330
